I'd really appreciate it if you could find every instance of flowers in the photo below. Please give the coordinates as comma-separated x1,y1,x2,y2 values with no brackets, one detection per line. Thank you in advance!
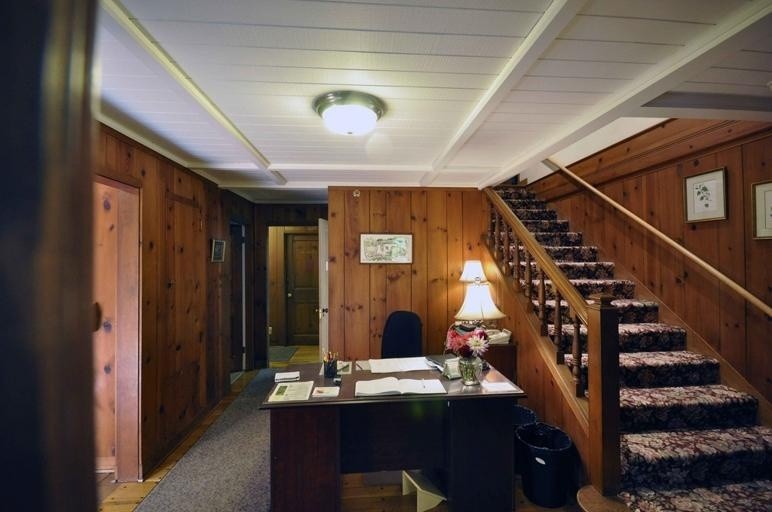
445,323,490,361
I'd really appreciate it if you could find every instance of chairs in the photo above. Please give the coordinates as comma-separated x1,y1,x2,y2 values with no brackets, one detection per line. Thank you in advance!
380,310,423,357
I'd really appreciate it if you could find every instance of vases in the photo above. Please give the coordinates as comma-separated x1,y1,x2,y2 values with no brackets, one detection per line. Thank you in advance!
458,356,483,386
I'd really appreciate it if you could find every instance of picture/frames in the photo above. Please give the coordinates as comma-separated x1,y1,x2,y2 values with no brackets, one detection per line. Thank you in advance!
749,178,772,240
681,166,727,226
359,232,413,264
211,239,226,263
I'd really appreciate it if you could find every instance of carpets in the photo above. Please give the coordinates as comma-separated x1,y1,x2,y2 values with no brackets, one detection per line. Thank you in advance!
489,183,772,511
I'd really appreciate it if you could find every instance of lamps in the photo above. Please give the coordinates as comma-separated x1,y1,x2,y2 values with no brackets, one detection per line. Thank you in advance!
312,89,384,137
454,259,507,321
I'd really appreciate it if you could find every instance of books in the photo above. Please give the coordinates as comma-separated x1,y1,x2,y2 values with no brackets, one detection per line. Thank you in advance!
354,376,448,398
311,386,340,398
268,381,315,402
427,353,457,373
369,356,438,374
275,371,300,383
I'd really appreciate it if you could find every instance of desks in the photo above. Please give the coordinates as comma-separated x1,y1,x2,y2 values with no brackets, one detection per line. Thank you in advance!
452,323,517,385
258,351,528,511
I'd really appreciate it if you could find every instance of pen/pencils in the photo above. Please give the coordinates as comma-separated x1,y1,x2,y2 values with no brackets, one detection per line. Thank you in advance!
322,349,338,378
355,363,363,370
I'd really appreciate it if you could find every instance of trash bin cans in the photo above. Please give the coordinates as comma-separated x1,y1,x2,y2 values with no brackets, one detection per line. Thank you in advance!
514,405,572,508
